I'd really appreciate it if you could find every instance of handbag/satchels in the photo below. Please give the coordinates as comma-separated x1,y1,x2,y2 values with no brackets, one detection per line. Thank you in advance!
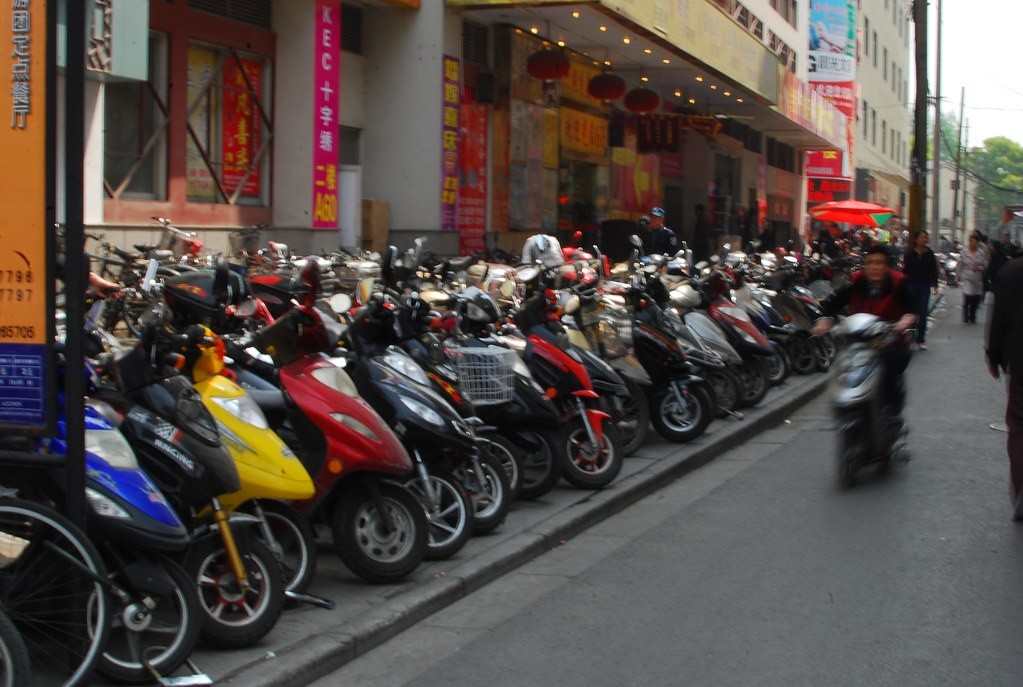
979,263,1004,291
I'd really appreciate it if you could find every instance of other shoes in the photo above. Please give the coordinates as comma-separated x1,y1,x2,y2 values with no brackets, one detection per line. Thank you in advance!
919,342,928,350
910,342,919,351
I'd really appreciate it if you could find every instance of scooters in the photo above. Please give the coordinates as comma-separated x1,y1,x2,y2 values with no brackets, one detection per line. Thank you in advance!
808,311,916,484
0,238,964,686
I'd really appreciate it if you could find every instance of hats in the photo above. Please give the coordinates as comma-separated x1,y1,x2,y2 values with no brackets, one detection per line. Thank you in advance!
650,207,665,218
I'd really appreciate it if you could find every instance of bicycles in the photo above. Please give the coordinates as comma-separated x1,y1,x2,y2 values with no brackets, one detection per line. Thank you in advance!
50,216,519,340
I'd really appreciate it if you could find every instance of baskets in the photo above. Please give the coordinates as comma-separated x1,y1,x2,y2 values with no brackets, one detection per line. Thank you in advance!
158,231,186,263
230,230,261,256
452,345,517,404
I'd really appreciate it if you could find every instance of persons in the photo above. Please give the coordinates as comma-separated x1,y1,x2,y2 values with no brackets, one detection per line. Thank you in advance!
905,229,937,349
639,207,677,257
991,208,1021,246
940,229,1023,323
818,223,909,260
694,204,713,258
758,218,772,250
521,234,564,265
810,246,920,418
984,258,1023,521
